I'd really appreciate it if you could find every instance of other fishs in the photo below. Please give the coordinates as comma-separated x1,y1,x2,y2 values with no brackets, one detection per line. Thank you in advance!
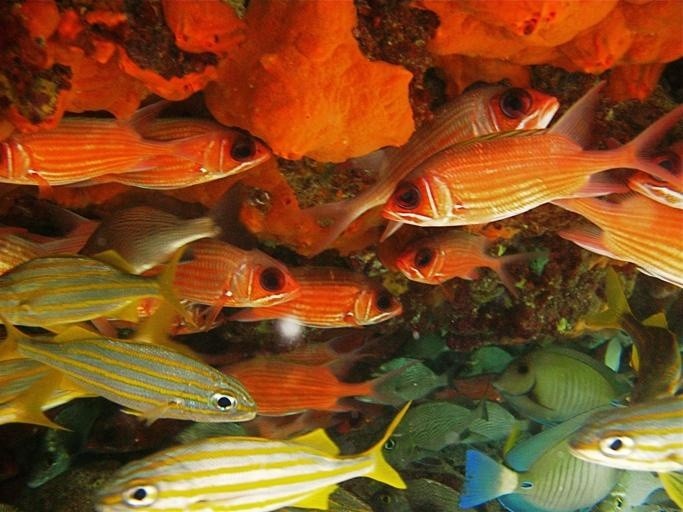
0,78,683,512
